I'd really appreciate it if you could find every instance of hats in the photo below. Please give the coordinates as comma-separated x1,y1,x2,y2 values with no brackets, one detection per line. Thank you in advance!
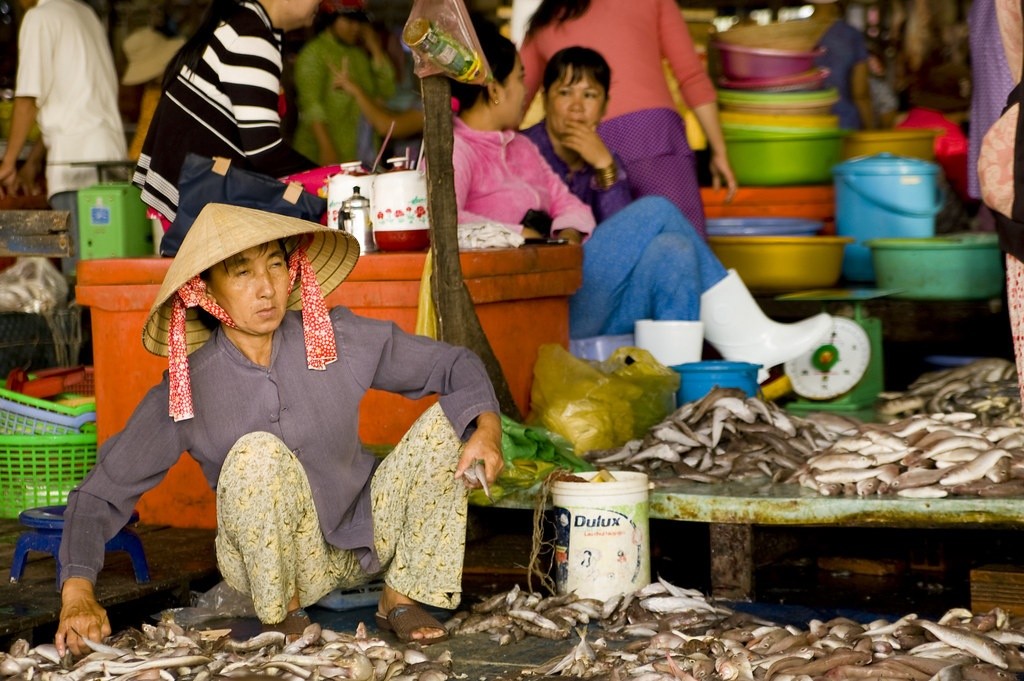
143,201,361,357
123,28,186,84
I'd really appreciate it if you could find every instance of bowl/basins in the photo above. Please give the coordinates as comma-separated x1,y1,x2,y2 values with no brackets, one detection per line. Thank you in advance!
860,231,1007,301
658,14,939,291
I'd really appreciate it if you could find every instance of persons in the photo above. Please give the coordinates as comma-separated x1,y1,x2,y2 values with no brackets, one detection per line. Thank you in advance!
513,46,634,244
1,0,512,281
960,0,1024,410
54,204,505,661
421,29,834,371
507,0,738,250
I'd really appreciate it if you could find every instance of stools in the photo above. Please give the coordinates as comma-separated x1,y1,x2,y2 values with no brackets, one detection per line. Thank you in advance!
7,504,153,596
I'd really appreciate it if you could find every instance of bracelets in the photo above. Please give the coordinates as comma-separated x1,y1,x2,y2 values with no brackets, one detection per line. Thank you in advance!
594,162,618,188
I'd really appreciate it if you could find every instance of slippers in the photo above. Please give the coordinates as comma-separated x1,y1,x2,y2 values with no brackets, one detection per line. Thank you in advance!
261,609,312,645
375,595,450,645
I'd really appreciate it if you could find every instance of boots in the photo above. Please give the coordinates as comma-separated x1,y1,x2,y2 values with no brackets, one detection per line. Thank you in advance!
634,320,704,369
698,267,833,386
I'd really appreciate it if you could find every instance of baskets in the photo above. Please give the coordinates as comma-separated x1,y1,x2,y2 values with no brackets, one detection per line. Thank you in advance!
0,359,97,519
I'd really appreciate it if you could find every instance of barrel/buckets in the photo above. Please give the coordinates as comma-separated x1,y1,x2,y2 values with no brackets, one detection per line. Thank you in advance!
672,359,763,418
834,148,945,284
544,472,651,608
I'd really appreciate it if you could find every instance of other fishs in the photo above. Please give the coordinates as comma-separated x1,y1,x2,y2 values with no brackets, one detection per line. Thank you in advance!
447,570,1024,681
589,363,1024,498
0,623,448,681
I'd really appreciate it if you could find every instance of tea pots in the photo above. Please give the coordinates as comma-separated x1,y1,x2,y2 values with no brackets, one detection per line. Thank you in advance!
337,186,381,257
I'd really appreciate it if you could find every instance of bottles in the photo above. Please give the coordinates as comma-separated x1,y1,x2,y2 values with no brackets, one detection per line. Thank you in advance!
403,17,493,86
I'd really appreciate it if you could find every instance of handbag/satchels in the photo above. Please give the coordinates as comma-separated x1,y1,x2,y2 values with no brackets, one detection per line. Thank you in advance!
524,341,681,457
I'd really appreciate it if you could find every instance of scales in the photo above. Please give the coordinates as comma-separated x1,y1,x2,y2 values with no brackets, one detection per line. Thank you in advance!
68,158,155,261
774,289,901,423
